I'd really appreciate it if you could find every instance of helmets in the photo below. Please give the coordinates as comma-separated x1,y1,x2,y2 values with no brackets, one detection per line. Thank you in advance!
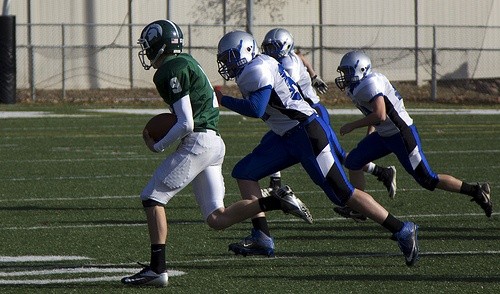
261,28,294,62
217,31,259,81
335,51,372,91
136,19,184,70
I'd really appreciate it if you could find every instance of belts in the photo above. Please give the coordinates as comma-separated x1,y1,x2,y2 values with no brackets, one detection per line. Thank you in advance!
193,127,219,136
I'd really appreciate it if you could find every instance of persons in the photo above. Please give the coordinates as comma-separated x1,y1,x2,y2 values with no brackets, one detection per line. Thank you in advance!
332,51,491,221
261,28,397,201
213,30,421,267
121,19,313,287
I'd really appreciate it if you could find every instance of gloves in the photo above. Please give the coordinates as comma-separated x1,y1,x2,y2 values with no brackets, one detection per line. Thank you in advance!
310,74,330,94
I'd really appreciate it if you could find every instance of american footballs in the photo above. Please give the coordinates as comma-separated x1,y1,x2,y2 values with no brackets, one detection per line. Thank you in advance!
143,113,178,143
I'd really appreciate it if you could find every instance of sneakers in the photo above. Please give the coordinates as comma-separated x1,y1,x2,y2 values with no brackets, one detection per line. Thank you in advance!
228,228,275,257
469,182,493,218
376,165,397,200
121,267,168,288
269,185,314,224
334,207,367,221
390,220,421,266
